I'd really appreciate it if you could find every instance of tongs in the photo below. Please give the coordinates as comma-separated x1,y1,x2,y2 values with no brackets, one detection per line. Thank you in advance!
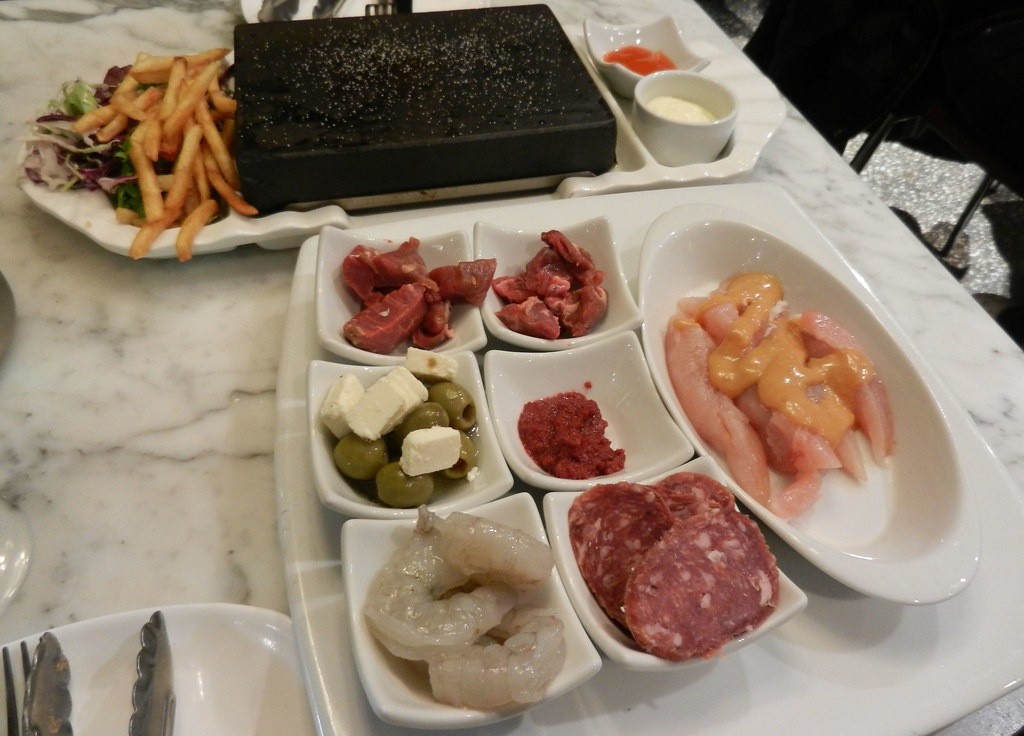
258,0,346,23
20,610,176,736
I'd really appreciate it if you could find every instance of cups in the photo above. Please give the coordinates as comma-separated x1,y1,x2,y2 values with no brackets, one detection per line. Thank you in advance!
632,69,740,167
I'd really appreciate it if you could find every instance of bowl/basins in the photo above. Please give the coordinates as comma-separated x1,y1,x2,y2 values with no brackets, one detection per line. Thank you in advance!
315,225,488,366
583,13,712,99
637,202,980,605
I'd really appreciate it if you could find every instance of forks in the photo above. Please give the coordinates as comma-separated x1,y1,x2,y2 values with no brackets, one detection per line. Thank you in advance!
365,0,398,18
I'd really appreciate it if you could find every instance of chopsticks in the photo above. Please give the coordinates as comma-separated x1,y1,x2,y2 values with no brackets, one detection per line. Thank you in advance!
2,641,31,736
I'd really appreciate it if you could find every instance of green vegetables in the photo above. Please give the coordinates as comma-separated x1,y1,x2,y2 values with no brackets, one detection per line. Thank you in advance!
15,63,147,218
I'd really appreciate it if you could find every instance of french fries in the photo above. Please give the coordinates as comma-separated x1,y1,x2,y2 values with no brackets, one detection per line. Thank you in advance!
71,48,258,262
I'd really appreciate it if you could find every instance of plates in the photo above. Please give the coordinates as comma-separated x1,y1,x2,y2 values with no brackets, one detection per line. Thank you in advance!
14,24,787,259
472,215,645,352
341,491,604,731
274,184,1023,736
483,331,695,490
542,455,809,673
0,600,317,736
306,352,514,519
240,0,490,17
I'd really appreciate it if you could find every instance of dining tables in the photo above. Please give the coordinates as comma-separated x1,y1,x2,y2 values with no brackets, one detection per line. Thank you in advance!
1,0,1024,736
